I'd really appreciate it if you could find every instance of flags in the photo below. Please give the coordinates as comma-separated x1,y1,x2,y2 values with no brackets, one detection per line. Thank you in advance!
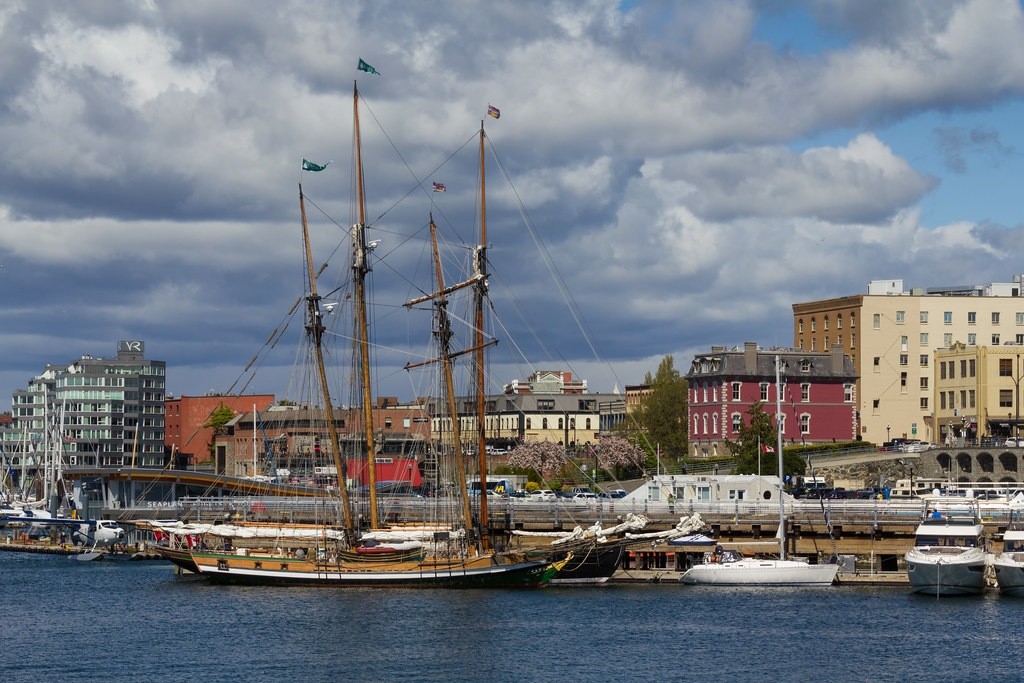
760,444,778,453
486,105,500,119
432,182,446,193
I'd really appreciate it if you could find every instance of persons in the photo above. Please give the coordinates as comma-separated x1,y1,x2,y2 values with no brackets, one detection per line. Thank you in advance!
313,548,339,562
877,492,883,504
366,539,376,547
60,530,67,548
667,494,674,515
946,436,949,448
295,547,304,559
144,541,148,553
856,490,860,499
928,509,941,518
205,540,209,548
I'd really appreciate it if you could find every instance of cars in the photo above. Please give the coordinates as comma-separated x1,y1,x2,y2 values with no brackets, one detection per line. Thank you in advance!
465,476,630,502
981,435,1002,448
789,482,890,499
881,438,940,453
1003,437,1024,448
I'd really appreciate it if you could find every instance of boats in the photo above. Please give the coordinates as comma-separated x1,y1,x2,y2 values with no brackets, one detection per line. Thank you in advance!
902,459,993,598
666,533,720,547
991,498,1024,599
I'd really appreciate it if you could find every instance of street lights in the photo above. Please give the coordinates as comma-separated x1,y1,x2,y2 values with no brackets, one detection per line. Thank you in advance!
961,414,966,448
886,424,891,442
594,444,601,485
908,462,915,500
948,420,954,447
714,462,720,475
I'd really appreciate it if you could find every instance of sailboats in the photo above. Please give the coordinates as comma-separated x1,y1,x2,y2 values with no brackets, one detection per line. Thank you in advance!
102,58,704,591
676,354,846,588
0,383,181,563
